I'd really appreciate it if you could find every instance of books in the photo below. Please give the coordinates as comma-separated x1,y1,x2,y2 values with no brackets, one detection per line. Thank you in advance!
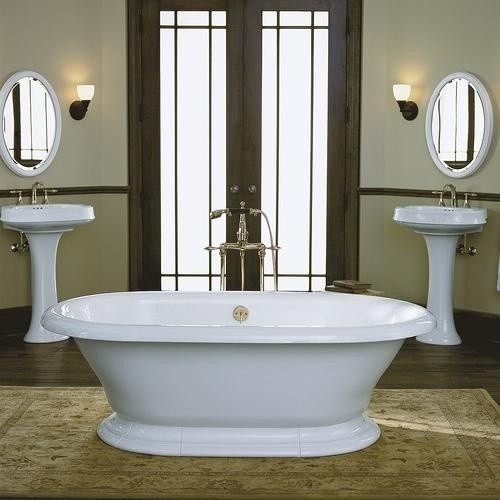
325,279,373,294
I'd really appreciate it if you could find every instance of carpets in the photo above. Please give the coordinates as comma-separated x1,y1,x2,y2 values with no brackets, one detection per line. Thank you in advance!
0,384,500,499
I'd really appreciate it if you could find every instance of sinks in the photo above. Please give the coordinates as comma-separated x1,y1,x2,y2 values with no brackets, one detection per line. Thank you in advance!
392,204,487,235
1,204,96,234
442,183,459,208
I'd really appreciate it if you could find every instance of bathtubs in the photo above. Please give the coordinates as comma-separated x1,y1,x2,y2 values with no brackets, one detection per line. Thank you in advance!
42,290,438,457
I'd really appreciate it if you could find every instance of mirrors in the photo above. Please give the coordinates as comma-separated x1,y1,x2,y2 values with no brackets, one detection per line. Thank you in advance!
0,69,64,180
424,73,494,180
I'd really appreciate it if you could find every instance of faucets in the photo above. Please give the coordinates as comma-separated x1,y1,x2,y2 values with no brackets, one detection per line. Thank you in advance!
29,182,44,205
238,220,247,243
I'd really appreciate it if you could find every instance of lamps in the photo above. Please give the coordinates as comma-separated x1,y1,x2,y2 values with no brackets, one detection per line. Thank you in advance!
69,84,95,121
393,84,418,121
